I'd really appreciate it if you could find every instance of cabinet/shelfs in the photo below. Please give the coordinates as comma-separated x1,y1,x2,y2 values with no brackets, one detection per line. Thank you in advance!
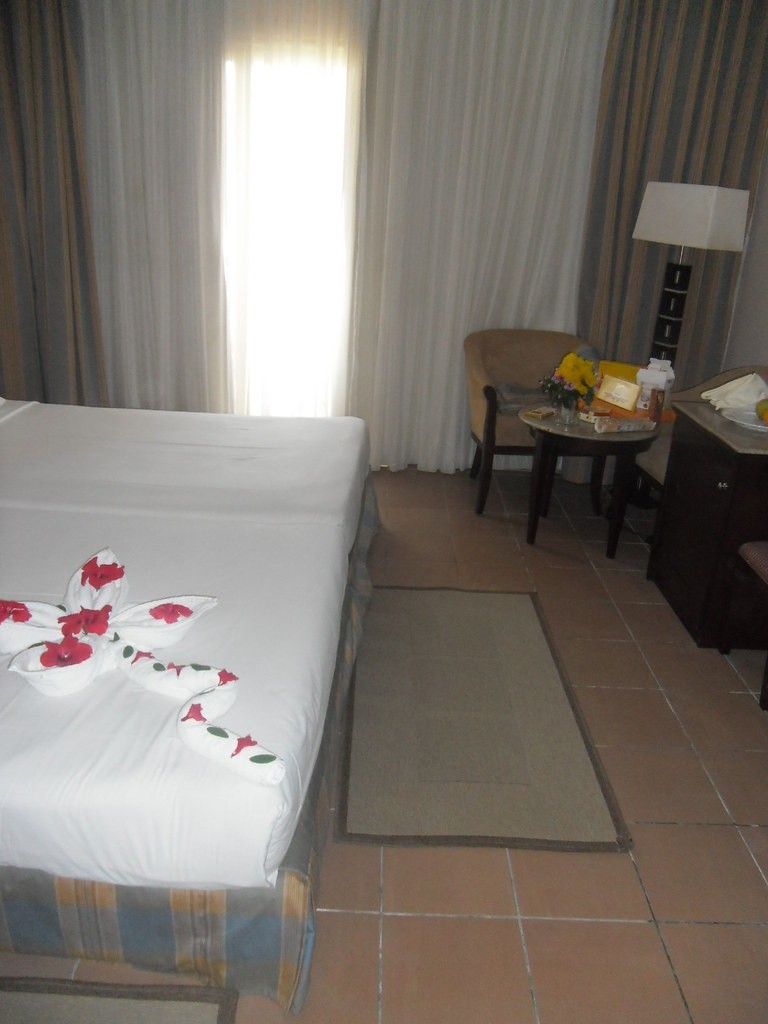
645,400,768,649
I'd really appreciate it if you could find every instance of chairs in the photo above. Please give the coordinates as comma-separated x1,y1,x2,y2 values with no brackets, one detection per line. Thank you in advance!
464,328,606,518
608,364,768,543
719,541,768,713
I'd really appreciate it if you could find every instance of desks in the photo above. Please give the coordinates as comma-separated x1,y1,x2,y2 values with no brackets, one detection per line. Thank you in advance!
518,402,659,558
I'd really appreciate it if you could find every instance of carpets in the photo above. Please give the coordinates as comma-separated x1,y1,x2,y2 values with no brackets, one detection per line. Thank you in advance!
329,584,632,852
0,974,239,1024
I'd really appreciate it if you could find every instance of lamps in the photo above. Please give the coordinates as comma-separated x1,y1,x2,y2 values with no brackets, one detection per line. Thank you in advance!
631,181,749,506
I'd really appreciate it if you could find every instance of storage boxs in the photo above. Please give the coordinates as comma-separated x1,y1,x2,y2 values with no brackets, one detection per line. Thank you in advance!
595,359,645,411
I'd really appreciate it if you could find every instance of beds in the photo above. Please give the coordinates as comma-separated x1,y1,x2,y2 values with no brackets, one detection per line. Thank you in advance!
0,397,385,1011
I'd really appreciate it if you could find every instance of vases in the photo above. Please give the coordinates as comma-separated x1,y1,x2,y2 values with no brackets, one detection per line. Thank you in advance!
556,397,580,424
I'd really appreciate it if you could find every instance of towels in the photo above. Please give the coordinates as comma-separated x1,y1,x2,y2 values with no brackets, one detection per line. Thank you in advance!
0,547,283,773
700,372,768,411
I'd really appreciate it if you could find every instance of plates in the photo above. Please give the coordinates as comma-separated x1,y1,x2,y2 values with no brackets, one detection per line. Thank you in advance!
721,408,768,432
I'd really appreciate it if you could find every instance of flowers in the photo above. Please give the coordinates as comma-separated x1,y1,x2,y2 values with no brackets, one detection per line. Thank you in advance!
0,547,276,775
539,352,605,411
